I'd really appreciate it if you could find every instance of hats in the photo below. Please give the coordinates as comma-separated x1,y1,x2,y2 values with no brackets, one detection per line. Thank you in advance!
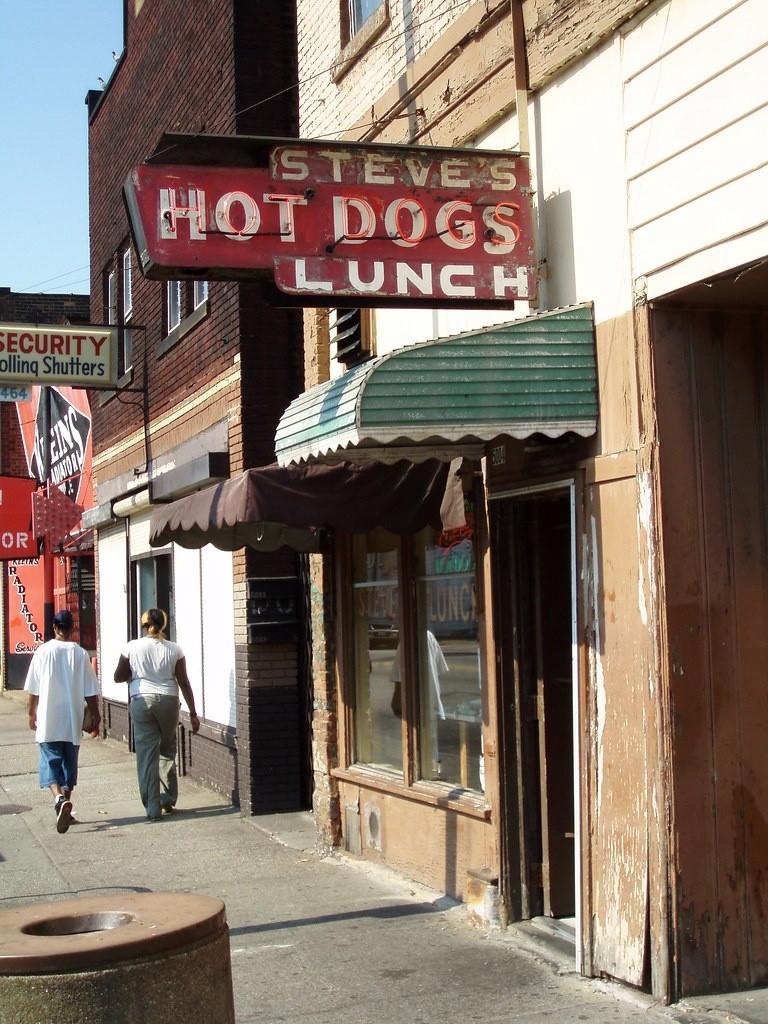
54,610,73,624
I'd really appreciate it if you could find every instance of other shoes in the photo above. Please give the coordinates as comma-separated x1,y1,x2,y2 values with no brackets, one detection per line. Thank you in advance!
148,813,160,821
55,796,73,834
68,814,75,825
162,804,172,815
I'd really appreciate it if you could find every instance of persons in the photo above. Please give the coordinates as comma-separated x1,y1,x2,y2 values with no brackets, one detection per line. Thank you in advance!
115,609,199,821
389,629,449,774
23,610,102,833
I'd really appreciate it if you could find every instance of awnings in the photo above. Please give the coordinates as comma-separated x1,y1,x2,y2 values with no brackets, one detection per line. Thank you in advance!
150,459,448,554
273,300,596,468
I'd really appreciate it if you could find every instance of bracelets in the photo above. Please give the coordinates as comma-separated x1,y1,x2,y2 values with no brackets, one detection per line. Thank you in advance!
190,713,197,717
